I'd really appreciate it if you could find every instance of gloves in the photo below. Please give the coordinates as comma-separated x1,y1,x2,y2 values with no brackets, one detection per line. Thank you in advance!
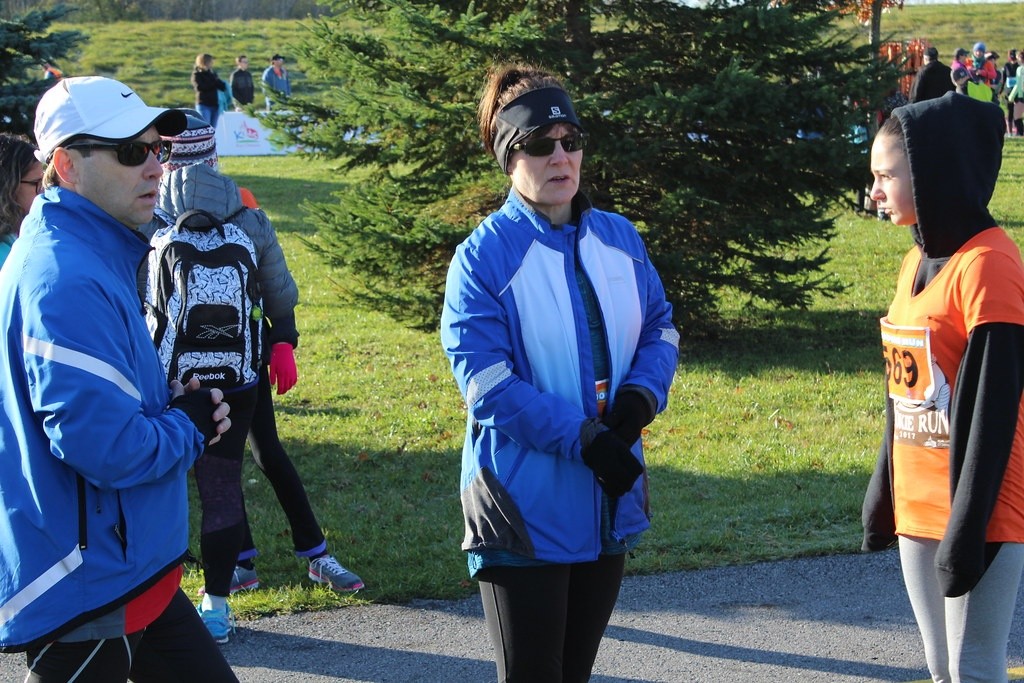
580,418,645,497
601,385,657,450
270,342,297,395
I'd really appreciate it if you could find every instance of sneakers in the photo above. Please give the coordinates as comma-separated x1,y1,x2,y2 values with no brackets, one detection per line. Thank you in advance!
194,602,236,644
309,555,364,591
198,566,259,597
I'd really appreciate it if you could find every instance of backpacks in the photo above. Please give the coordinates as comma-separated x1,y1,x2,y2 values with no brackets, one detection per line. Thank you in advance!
145,205,263,395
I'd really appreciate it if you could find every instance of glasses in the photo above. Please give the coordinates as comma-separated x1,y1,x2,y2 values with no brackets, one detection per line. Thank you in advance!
512,132,590,156
20,178,44,195
46,140,172,166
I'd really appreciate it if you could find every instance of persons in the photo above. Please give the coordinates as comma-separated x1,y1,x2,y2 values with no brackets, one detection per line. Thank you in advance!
862,91,1024,683
0,52,365,683
796,43,1024,220
439,60,681,683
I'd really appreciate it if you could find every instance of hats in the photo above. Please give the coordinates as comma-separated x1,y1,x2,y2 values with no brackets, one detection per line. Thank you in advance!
272,54,285,60
33,75,188,168
923,47,938,57
159,107,218,186
973,42,985,52
955,48,970,56
